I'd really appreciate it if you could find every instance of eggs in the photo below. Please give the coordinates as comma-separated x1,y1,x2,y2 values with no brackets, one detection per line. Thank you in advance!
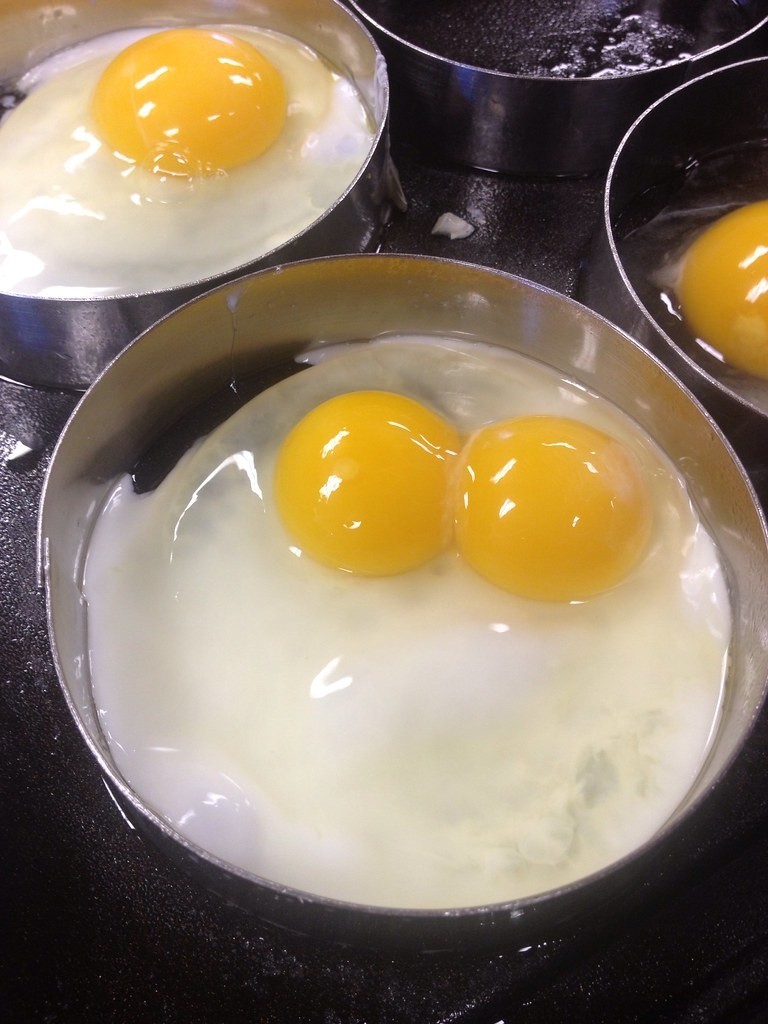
0,21,768,913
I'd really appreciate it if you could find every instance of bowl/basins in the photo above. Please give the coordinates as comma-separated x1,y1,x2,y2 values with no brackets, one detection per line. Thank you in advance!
0,0,388,388
574,57,768,501
36,254,768,949
349,0,768,176
